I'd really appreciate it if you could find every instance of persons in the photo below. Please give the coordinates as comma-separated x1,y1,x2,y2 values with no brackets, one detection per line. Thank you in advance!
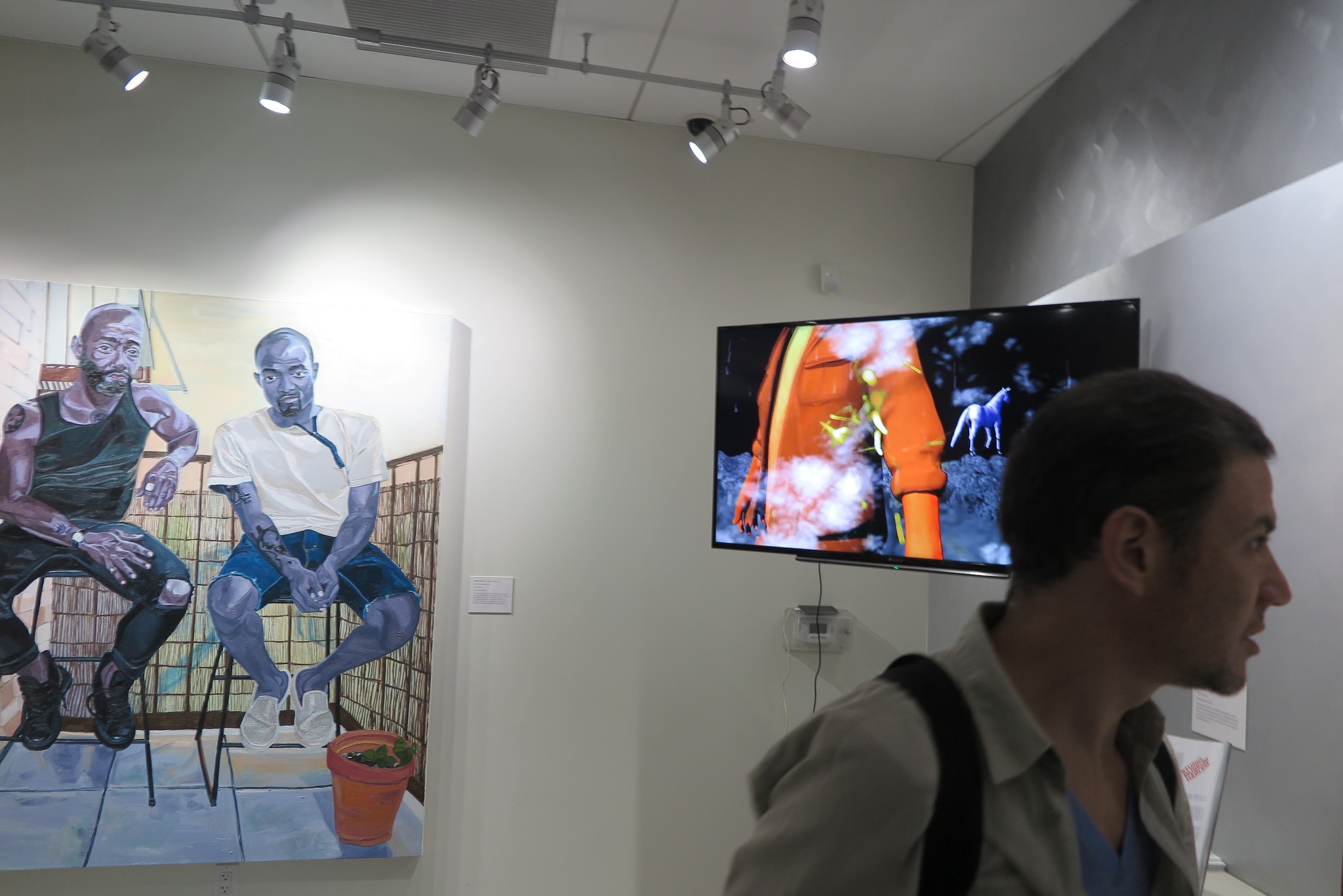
732,318,950,562
720,371,1293,896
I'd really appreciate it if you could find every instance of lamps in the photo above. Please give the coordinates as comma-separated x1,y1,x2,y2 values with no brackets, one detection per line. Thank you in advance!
688,97,753,164
259,26,301,115
759,60,812,138
81,5,150,91
781,0,826,70
454,55,501,138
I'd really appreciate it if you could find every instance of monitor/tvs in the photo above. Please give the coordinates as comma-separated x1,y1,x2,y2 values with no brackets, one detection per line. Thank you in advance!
711,298,1142,579
